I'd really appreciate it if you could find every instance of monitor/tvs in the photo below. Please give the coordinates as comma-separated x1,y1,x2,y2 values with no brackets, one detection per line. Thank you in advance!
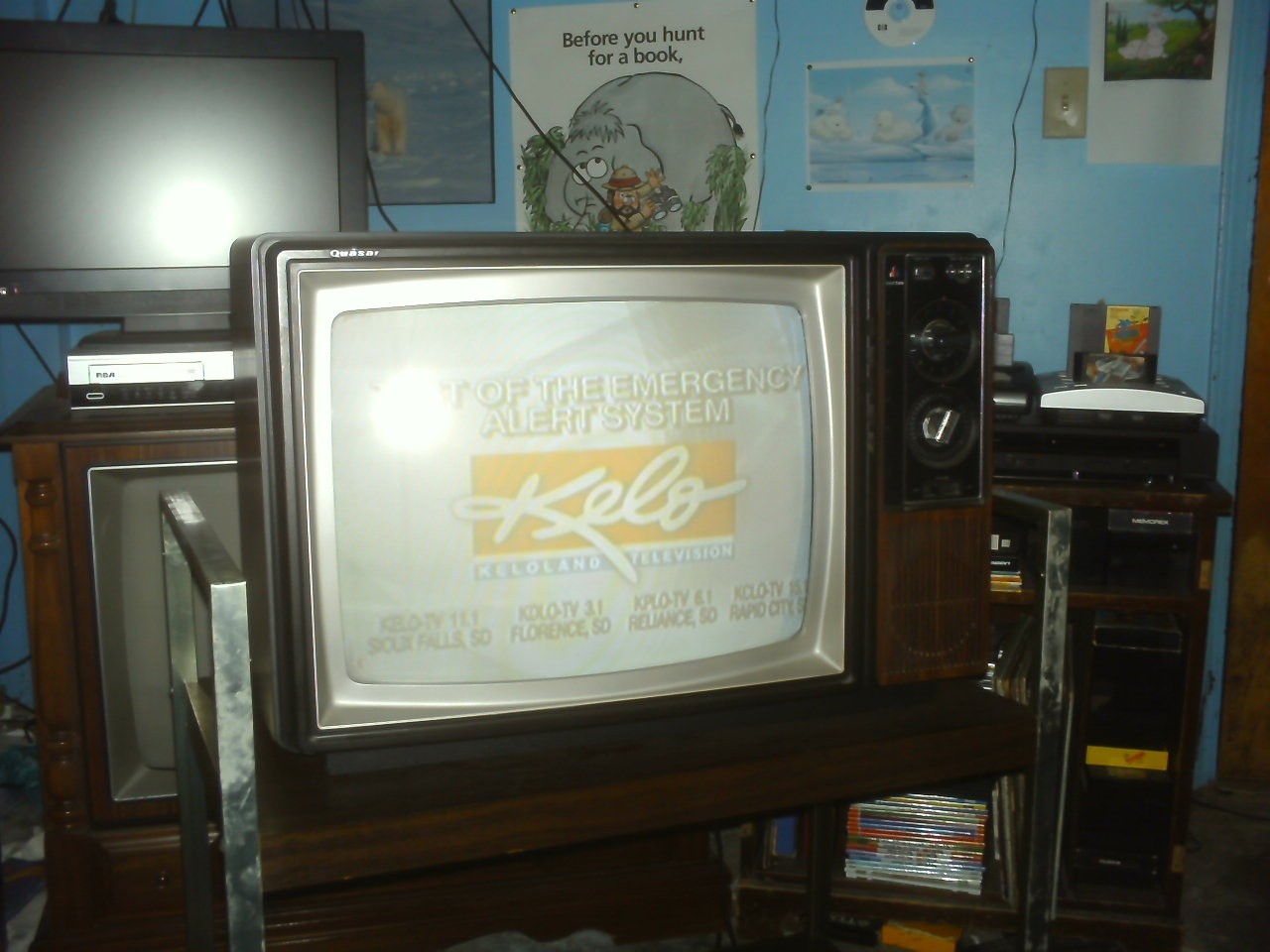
229,229,1000,799
0,16,367,320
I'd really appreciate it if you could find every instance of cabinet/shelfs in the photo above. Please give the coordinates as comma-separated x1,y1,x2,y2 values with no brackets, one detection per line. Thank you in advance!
998,472,1235,870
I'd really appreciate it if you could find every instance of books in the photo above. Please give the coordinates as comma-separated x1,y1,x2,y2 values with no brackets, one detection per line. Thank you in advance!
759,771,1040,905
989,530,1025,593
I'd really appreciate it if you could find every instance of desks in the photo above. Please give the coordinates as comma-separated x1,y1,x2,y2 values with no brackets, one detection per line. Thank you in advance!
5,384,228,951
256,691,1046,951
